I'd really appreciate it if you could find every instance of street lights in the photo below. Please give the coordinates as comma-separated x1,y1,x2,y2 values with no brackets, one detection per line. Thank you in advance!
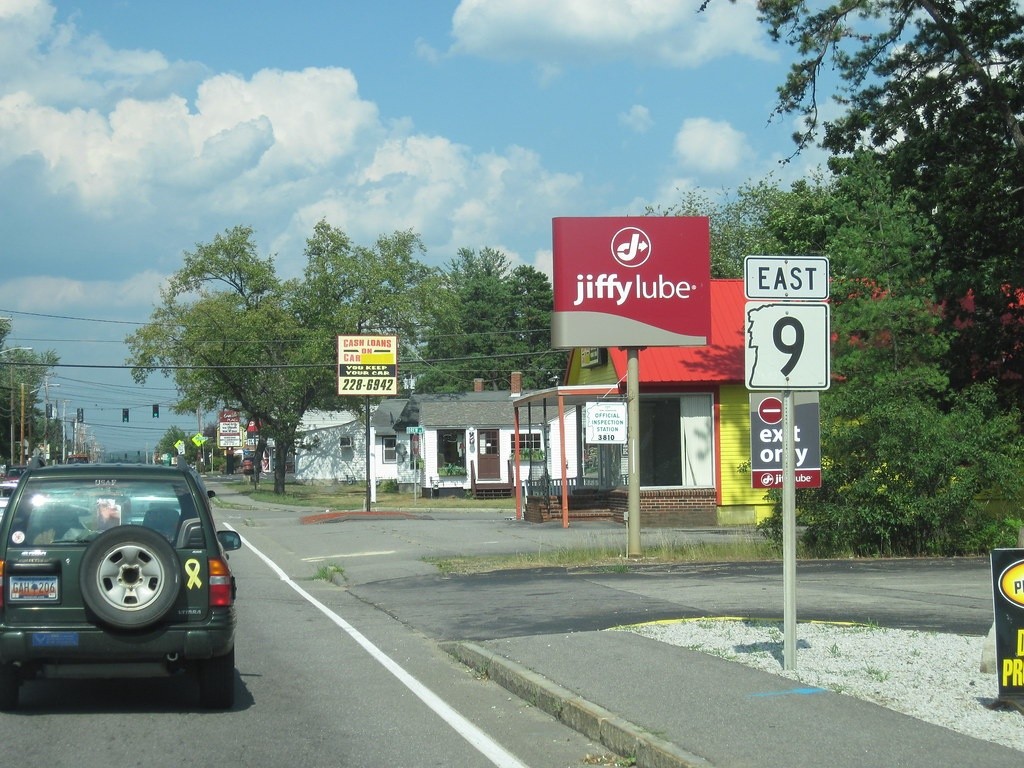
27,382,61,464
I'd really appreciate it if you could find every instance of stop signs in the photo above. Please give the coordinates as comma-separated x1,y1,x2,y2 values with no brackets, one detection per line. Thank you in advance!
757,397,783,426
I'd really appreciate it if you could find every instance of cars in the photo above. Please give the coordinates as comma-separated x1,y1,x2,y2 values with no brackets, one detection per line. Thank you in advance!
0,478,26,528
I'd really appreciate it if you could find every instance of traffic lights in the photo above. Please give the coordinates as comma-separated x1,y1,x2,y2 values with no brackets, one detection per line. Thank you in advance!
46,404,52,418
122,408,129,423
153,404,159,418
77,408,83,422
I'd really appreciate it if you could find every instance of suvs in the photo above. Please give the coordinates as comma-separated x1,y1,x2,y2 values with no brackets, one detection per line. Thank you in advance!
3,461,242,712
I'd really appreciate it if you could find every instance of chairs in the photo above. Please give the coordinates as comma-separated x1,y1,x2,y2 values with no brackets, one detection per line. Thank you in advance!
26,505,86,539
141,508,179,534
